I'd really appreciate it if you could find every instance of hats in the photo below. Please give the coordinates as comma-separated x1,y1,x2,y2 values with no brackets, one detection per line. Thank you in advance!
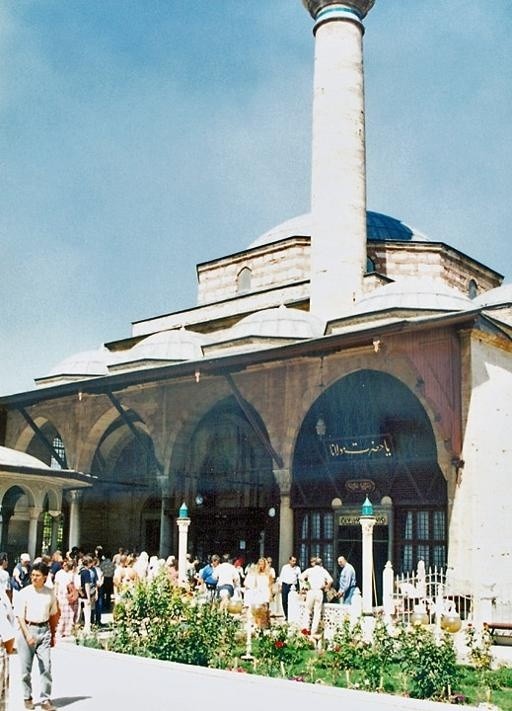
20,552,30,561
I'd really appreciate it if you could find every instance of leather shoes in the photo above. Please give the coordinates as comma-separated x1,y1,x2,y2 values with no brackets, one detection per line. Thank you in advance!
41,699,54,711
24,698,34,709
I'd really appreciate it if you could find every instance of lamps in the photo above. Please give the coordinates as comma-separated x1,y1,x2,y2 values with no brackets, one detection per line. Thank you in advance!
314,346,327,440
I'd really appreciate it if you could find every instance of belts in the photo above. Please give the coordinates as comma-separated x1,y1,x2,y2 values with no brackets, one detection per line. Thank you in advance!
24,619,49,627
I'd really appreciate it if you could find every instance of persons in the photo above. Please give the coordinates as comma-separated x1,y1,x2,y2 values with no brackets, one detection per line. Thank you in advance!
14,562,59,710
1,589,16,710
101,547,355,636
1,545,105,641
